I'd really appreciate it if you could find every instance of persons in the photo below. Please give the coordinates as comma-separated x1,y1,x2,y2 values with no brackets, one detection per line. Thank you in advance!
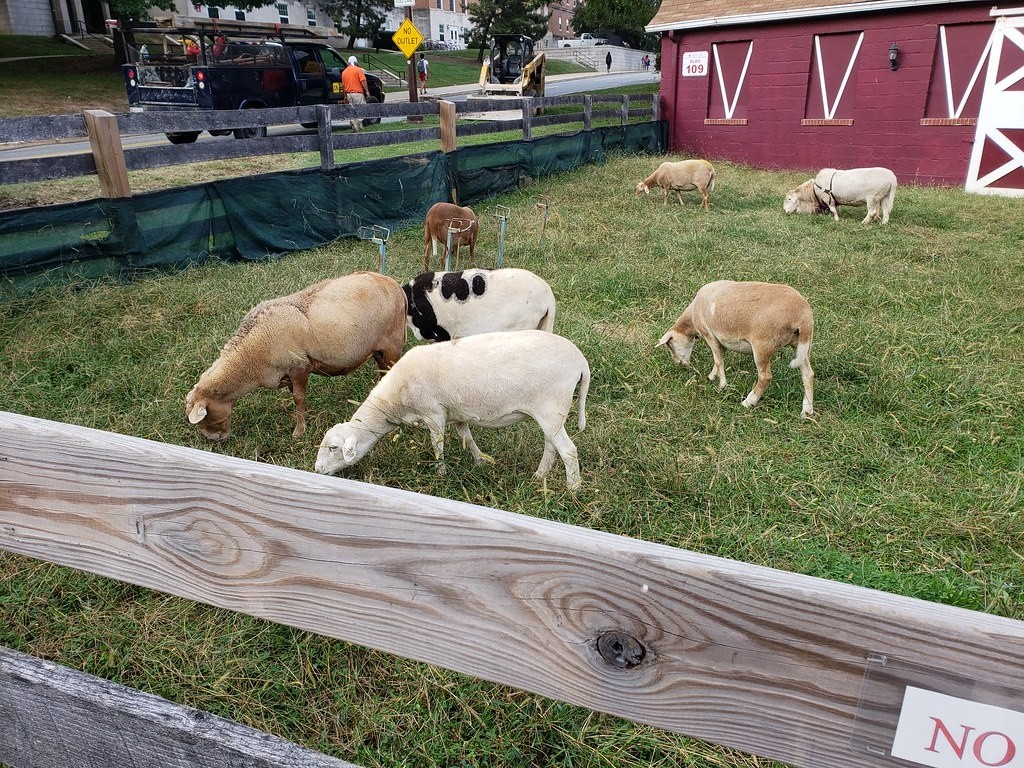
416,53,430,94
342,56,369,133
641,56,645,70
645,55,650,71
606,51,612,73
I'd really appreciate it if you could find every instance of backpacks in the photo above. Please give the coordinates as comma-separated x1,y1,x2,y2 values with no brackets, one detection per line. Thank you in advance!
418,59,425,73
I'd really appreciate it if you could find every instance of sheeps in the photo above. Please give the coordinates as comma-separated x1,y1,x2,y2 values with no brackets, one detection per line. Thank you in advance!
783,167,898,224
424,202,479,274
184,269,408,443
316,328,592,493
635,158,717,211
654,280,816,420
403,269,557,341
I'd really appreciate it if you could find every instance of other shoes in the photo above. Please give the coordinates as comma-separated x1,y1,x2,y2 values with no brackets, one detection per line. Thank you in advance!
350,119,359,133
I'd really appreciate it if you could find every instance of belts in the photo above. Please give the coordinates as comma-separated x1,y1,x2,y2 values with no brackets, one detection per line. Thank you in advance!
347,92,362,93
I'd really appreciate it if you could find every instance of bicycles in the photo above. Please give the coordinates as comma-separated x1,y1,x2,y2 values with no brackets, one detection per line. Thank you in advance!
423,39,461,51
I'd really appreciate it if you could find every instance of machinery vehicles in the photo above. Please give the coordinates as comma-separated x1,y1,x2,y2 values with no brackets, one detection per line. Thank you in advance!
456,34,547,121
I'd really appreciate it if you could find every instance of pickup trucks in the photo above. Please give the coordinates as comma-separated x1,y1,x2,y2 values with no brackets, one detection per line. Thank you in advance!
556,33,607,48
110,15,385,144
